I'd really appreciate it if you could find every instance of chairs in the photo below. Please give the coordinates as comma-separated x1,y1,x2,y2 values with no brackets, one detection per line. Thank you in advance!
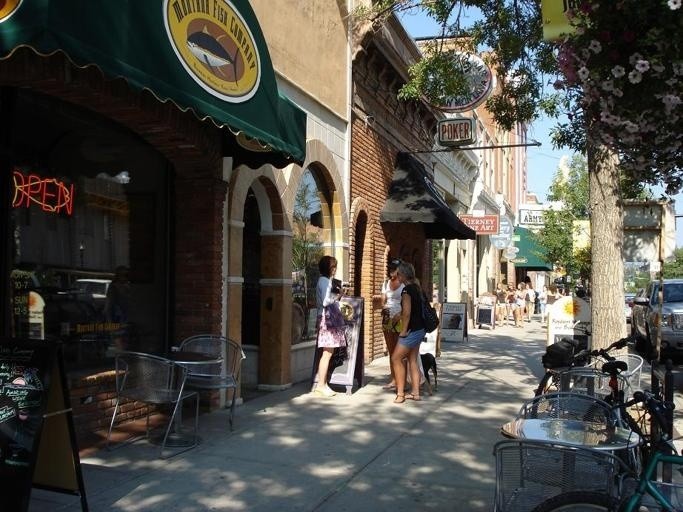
105,351,200,459
596,354,643,401
517,391,624,489
492,439,619,512
177,333,246,432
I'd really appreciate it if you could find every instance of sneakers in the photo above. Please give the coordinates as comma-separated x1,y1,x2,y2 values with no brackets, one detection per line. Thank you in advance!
311,383,336,397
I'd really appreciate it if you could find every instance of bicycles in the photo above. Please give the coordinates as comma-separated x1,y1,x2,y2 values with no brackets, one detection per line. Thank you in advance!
489,434,682,512
531,323,590,418
579,335,682,480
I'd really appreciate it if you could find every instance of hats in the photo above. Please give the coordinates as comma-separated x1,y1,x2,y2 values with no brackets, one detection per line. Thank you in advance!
386,258,400,268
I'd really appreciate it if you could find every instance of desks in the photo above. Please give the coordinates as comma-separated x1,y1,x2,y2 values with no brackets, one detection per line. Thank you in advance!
146,352,224,447
501,419,644,495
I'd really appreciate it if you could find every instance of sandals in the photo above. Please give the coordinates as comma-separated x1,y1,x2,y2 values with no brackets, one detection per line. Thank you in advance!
382,381,424,403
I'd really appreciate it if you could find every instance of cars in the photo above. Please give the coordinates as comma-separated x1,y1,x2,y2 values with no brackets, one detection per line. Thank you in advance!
631,279,683,353
624,293,635,324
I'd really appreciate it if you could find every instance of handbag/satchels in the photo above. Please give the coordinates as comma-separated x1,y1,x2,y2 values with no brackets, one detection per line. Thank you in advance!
325,302,344,327
422,304,440,333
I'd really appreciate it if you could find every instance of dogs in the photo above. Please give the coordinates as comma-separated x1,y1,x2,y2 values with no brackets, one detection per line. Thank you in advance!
420,353,438,396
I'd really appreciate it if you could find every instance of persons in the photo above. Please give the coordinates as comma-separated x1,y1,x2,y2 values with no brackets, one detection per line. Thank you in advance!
312,256,346,397
495,281,562,327
380,258,405,390
391,262,426,403
